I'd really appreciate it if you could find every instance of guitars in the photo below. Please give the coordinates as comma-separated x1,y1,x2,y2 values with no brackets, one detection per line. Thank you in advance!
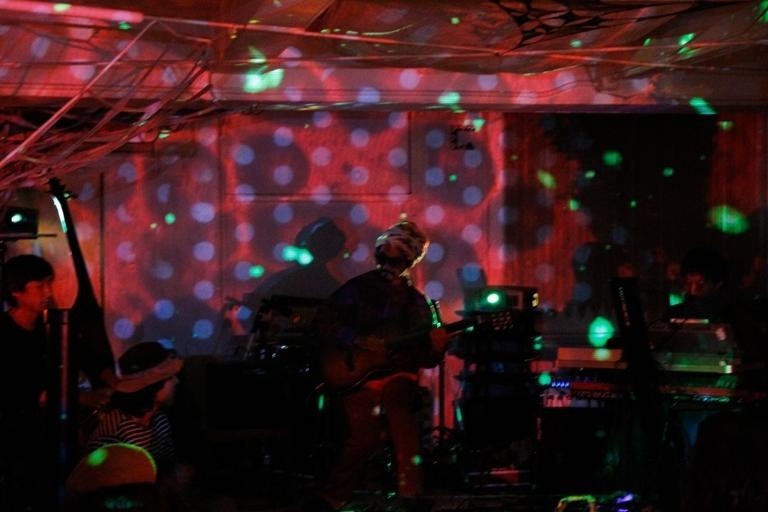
304,311,515,400
44,179,115,386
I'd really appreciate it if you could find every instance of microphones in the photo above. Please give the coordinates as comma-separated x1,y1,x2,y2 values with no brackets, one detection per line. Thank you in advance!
427,298,445,328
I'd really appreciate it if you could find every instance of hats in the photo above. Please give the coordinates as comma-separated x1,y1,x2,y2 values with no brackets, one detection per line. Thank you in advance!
294,217,345,264
107,340,183,392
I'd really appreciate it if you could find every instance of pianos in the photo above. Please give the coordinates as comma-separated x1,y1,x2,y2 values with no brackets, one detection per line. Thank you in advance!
528,331,739,409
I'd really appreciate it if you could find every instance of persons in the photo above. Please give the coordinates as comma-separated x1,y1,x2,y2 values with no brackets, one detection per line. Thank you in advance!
1,222,766,512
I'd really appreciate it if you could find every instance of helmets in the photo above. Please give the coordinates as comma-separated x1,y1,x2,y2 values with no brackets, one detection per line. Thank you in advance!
373,221,429,265
